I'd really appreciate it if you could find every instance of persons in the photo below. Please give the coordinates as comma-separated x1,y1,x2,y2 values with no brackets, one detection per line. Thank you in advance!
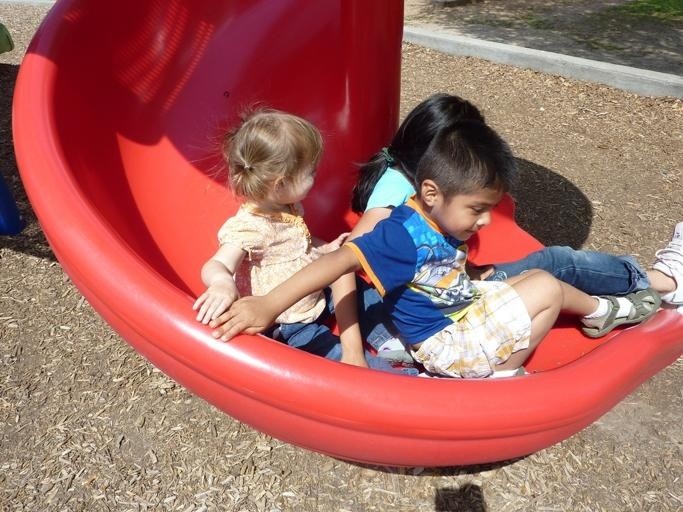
191,106,421,376
329,92,683,370
202,119,667,381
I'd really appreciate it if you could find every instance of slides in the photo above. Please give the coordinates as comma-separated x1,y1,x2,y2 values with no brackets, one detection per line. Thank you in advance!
11,0,683,468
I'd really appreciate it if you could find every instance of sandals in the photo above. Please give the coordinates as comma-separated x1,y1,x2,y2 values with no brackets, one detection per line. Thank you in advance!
577,286,664,339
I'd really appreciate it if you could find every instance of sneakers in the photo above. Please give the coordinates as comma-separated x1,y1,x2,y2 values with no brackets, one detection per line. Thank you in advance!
650,220,683,306
